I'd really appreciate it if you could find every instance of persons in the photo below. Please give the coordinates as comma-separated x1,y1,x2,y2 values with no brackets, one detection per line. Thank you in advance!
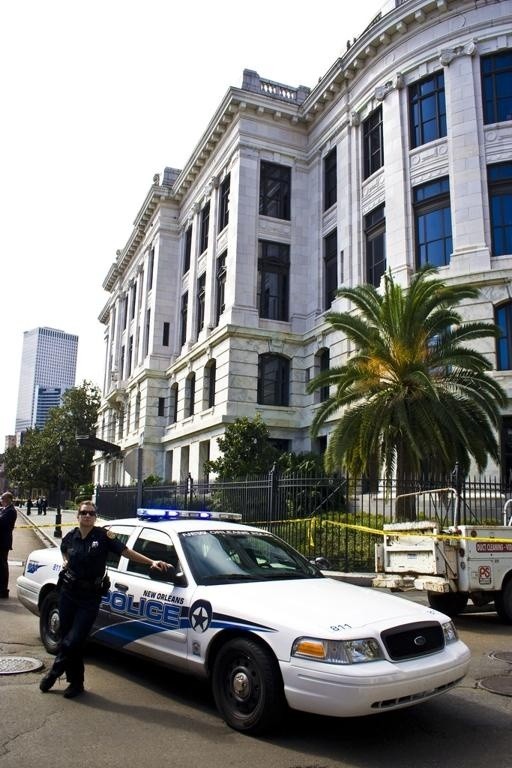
37,495,43,515
37,500,174,698
41,496,48,516
0,491,17,599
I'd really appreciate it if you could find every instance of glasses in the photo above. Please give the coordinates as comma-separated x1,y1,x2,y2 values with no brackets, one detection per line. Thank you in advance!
79,510,96,517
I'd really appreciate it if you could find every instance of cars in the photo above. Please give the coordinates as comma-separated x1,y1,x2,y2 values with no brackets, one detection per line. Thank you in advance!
13,507,476,731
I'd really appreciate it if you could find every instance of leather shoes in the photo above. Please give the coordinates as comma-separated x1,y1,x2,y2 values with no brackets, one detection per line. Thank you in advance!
63,683,84,697
40,670,57,692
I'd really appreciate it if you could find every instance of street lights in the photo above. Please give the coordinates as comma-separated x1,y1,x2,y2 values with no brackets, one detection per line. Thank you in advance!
53,435,67,539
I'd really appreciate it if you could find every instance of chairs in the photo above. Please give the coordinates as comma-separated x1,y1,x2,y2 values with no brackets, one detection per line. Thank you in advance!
141,536,251,586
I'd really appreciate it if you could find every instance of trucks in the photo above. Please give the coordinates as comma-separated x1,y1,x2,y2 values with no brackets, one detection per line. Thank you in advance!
368,486,512,625
